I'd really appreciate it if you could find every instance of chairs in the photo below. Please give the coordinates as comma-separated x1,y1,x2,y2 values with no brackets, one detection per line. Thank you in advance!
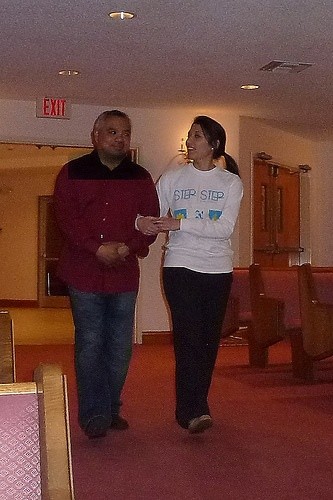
290,263,333,377
220,266,251,341
249,264,300,371
0,360,74,500
0,310,16,383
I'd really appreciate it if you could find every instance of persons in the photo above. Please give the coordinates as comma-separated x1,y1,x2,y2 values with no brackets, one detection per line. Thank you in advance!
135,116,244,432
46,110,160,439
